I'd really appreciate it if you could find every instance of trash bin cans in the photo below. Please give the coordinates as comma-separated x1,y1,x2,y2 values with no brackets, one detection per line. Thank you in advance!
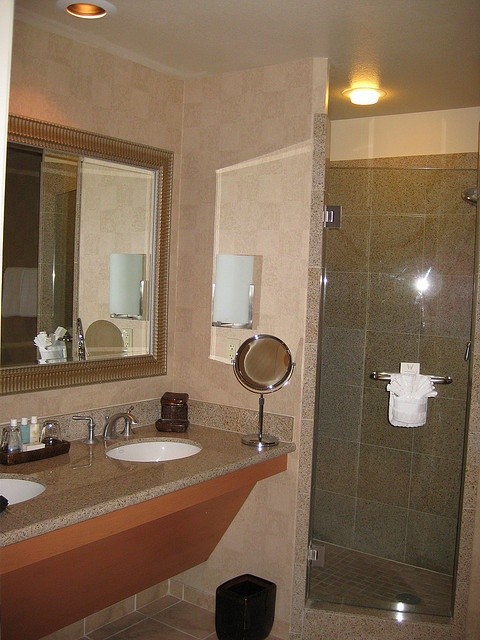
215,574,276,640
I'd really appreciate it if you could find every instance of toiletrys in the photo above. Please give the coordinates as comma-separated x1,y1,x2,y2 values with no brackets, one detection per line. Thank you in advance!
19,418,30,444
29,416,39,444
8,419,21,455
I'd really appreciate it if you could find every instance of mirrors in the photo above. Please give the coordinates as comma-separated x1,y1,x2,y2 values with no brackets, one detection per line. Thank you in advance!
232,333,296,448
85,320,124,349
0,114,175,397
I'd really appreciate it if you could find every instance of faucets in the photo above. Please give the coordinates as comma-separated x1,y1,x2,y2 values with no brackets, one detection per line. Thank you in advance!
103,412,138,443
75,314,87,361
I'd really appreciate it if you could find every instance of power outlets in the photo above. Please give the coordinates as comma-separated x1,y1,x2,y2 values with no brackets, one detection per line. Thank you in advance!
225,338,241,363
122,329,133,348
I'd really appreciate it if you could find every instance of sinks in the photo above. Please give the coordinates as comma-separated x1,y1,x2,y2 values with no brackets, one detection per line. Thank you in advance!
107,439,204,463
0,472,46,507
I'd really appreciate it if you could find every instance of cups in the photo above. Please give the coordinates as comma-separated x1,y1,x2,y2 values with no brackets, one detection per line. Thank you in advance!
40,420,62,448
0,426,23,455
